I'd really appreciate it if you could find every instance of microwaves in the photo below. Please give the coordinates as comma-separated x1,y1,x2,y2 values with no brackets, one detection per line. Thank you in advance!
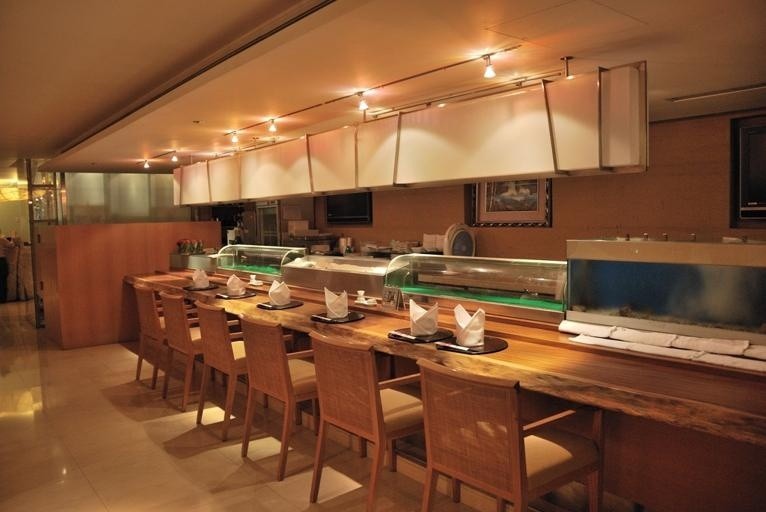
326,191,373,226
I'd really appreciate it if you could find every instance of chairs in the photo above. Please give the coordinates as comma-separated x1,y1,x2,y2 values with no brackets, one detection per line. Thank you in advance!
159,291,249,412
133,284,215,391
308,330,459,512
194,299,301,442
238,313,367,481
415,357,602,512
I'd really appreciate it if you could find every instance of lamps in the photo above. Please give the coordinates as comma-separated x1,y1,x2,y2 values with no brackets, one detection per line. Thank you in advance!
231,131,239,143
267,118,276,131
357,92,369,109
483,56,496,78
170,152,177,161
143,160,149,168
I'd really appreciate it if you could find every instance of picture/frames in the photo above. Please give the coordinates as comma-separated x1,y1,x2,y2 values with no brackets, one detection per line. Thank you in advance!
469,178,553,228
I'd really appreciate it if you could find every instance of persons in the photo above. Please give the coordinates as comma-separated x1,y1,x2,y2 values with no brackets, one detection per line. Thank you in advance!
0,231,16,305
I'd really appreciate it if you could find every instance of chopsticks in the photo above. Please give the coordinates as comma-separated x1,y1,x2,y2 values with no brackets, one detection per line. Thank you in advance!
258,301,277,309
311,313,338,324
216,292,231,299
387,329,433,344
433,340,478,353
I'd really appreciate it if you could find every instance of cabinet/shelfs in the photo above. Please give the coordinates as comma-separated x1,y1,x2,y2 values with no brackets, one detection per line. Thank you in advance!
383,254,567,325
215,244,305,284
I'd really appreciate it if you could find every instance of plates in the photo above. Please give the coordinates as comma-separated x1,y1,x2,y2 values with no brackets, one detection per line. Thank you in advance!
388,327,454,345
310,311,367,324
215,291,256,300
183,283,220,292
257,300,305,311
434,336,509,355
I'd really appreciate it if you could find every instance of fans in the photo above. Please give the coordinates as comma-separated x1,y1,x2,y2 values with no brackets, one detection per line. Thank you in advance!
440,222,475,275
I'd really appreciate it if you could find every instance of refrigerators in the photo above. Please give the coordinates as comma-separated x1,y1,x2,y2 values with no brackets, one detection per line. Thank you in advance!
256,200,279,244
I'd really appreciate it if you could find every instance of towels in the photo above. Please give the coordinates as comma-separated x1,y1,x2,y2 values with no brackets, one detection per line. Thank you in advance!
453,304,486,348
269,279,292,307
324,287,349,319
409,298,438,338
226,274,246,297
193,269,210,289
